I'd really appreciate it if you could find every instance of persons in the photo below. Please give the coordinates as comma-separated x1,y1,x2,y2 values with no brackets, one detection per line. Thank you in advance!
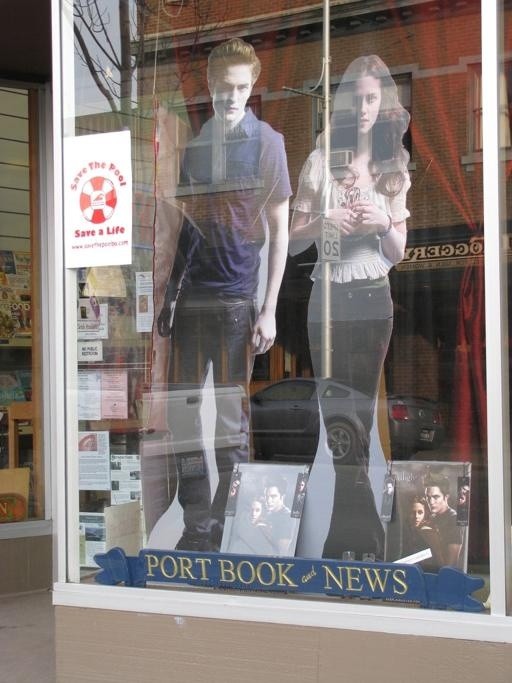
379,473,393,516
294,475,305,517
422,475,463,564
156,38,291,555
460,480,469,530
288,53,413,561
405,497,443,568
259,486,292,543
238,499,269,545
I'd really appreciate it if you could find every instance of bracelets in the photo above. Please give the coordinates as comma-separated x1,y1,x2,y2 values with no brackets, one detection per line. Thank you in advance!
377,213,393,237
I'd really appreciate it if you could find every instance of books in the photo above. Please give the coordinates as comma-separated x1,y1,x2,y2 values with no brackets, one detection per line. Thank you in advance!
220,462,309,557
384,460,472,573
0,247,33,521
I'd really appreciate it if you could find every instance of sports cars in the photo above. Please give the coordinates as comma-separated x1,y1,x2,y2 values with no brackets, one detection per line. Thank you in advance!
250,376,444,463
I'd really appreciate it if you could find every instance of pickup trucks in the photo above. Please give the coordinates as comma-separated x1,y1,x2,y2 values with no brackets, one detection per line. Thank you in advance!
79,382,249,509
